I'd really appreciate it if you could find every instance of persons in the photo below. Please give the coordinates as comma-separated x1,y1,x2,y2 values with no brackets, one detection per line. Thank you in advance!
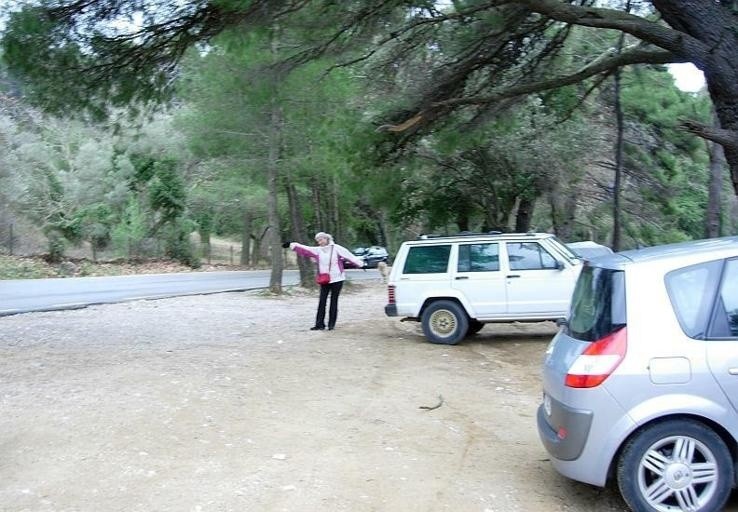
282,232,367,330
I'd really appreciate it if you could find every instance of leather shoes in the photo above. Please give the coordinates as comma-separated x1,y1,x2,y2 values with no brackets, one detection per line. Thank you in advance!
311,326,323,330
328,324,333,330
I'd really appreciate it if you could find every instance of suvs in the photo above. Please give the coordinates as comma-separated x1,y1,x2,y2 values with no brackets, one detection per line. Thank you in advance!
344,246,389,268
385,230,583,345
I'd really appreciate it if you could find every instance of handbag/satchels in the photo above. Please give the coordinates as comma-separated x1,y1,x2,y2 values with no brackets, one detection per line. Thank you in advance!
316,273,330,285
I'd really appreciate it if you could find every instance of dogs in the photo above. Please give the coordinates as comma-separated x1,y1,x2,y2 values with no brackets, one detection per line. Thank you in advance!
377,262,391,282
